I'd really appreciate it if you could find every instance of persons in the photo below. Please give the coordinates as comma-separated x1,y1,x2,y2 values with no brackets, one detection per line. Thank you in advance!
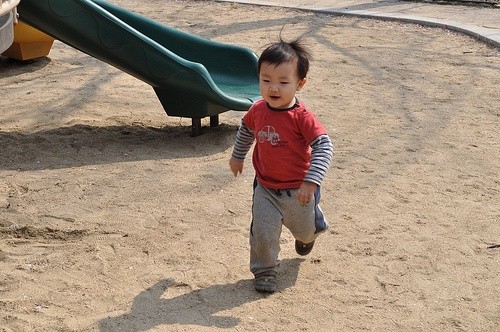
228,39,334,293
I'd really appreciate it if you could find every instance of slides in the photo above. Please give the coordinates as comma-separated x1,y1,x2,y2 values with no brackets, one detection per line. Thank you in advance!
17,0,266,119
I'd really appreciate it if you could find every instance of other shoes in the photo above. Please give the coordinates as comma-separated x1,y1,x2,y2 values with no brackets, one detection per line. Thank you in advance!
295,239,315,256
253,274,277,292
